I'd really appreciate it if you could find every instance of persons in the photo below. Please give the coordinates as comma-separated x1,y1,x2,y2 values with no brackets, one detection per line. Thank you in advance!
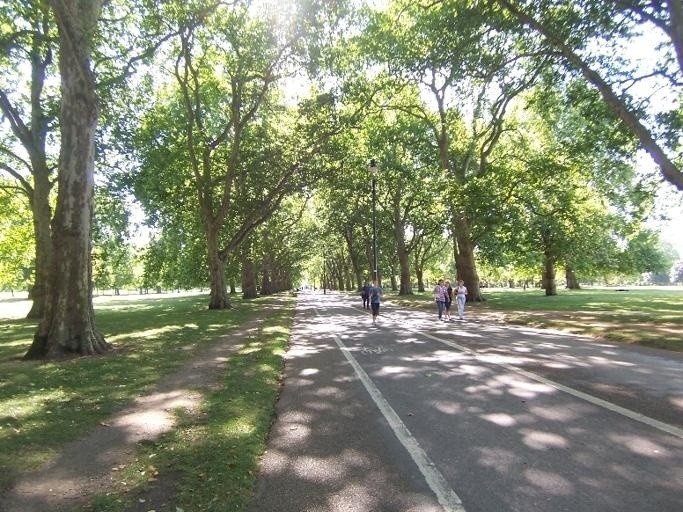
359,281,369,310
367,281,383,326
443,280,452,318
452,280,468,320
431,279,449,320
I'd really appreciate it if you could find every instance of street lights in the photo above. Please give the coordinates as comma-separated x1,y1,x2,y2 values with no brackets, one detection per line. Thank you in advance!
367,159,381,287
